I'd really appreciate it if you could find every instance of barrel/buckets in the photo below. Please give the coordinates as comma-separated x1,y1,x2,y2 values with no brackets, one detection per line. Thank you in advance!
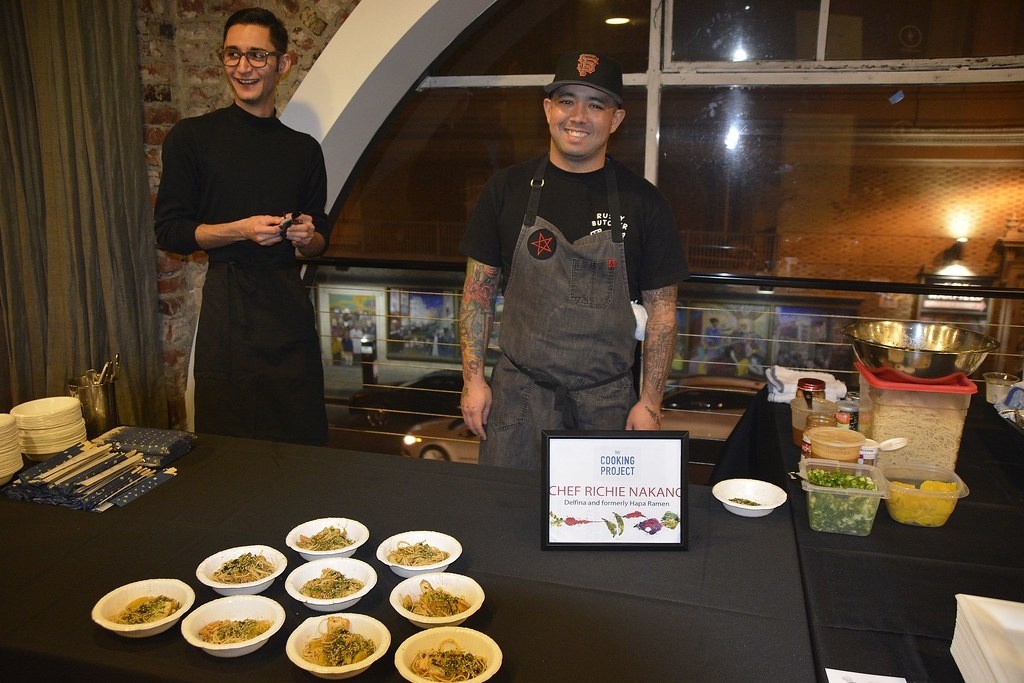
791,397,837,447
66,374,118,441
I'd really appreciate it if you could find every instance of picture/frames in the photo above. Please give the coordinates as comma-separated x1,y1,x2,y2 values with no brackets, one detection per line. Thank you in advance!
539,430,689,550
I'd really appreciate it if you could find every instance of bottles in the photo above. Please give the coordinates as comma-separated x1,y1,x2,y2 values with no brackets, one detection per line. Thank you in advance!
801,414,837,458
856,439,880,467
803,425,866,462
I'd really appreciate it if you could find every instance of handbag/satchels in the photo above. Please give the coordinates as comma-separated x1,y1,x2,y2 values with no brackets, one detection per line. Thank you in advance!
709,383,801,488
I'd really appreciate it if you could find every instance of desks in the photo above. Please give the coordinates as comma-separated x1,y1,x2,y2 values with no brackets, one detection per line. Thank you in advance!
0,384,1024,683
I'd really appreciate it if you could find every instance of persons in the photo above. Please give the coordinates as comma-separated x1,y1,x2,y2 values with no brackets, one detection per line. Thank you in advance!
340,320,362,368
151,6,334,450
456,49,691,473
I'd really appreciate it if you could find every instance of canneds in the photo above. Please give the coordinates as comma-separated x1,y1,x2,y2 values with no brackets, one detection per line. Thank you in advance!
800,400,908,466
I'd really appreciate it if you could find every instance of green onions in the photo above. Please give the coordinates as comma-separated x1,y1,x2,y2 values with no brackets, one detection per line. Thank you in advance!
325,628,373,667
418,588,462,617
807,469,878,537
432,651,482,677
127,595,172,621
306,572,350,598
218,621,249,638
401,544,439,560
308,531,348,550
220,553,262,576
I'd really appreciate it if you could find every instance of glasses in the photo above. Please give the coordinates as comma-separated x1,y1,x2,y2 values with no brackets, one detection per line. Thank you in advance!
217,44,284,68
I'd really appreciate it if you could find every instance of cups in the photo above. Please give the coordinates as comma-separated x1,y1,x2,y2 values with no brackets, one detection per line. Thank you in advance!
983,372,1020,404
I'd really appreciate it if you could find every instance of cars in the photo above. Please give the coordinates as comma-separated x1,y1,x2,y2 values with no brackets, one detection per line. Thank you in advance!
655,374,768,440
399,414,484,465
346,370,491,431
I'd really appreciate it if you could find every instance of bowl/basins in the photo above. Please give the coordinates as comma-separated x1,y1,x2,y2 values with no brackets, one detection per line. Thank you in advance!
394,626,503,683
92,579,196,638
712,478,788,517
196,545,287,596
879,463,969,526
286,613,391,680
377,531,462,578
285,558,377,612
389,573,485,629
0,396,88,486
286,517,369,562
181,595,286,657
840,322,1000,383
798,458,890,536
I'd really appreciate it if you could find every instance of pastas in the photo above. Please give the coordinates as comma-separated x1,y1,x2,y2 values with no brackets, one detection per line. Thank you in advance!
111,595,178,625
867,394,967,474
389,539,449,565
198,618,270,644
300,569,364,599
399,582,472,618
295,527,352,551
413,639,488,683
211,554,275,584
299,618,376,667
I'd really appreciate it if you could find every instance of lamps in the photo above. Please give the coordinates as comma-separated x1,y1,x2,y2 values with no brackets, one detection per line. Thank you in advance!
953,236,968,262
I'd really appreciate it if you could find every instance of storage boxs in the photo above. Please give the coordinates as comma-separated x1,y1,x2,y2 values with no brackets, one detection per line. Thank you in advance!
854,361,978,482
799,458,888,536
880,463,969,526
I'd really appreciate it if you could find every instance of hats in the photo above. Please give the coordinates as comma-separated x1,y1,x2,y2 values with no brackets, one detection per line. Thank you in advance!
542,46,627,105
